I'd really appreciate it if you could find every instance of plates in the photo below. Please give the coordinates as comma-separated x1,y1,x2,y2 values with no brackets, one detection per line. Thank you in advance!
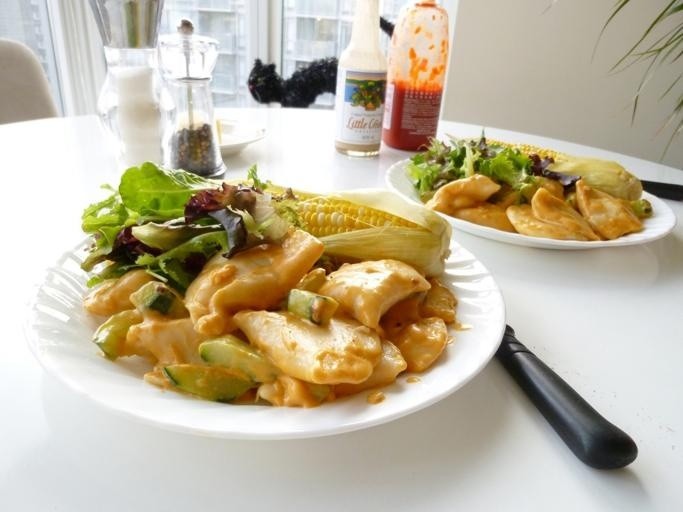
388,152,676,246
28,189,507,436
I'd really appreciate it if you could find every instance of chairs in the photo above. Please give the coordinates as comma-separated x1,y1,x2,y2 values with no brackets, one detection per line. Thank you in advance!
1,38,59,125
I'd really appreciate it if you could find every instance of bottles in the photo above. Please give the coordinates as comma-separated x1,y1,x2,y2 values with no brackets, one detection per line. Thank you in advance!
92,1,227,179
334,1,450,158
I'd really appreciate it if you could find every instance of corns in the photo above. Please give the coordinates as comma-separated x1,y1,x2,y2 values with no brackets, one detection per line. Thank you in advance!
293,189,454,276
463,135,644,202
